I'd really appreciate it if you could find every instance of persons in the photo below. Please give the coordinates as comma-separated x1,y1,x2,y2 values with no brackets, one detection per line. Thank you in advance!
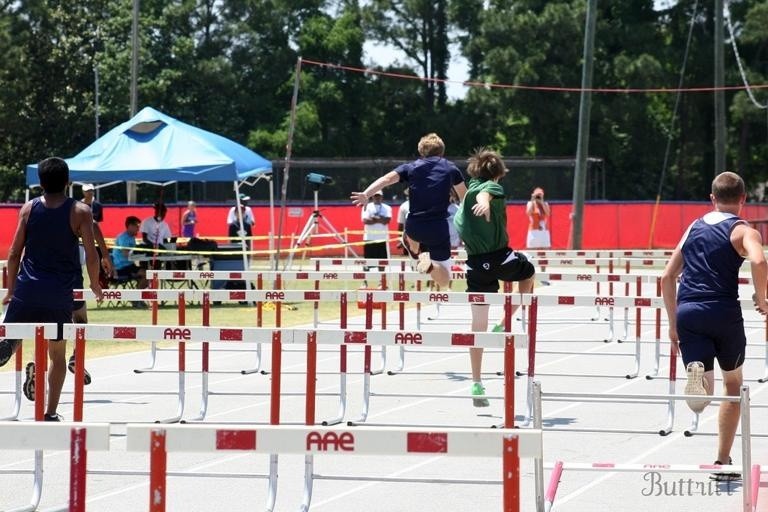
524,187,553,285
74,183,101,222
351,130,468,289
139,202,174,269
659,170,768,477
360,187,392,290
453,145,537,408
443,188,463,250
228,203,252,245
395,185,412,255
113,217,151,309
20,220,112,402
0,155,104,422
180,200,198,237
226,193,256,226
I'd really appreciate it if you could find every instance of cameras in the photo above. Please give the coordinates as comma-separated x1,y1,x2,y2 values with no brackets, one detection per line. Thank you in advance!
535,195,541,199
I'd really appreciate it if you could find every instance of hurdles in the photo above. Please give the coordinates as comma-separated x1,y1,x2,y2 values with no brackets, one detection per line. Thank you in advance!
1,249,768,512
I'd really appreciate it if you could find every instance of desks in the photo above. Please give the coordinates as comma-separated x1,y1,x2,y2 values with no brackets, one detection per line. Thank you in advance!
128,250,204,306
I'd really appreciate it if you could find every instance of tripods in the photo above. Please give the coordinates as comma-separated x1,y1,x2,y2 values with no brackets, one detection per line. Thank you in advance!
283,190,359,263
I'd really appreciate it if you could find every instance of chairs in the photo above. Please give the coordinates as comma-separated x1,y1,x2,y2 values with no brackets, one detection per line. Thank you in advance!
96,247,137,309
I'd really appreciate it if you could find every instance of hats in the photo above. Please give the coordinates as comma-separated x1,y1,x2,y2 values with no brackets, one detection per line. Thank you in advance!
373,190,383,196
82,183,94,191
239,194,251,201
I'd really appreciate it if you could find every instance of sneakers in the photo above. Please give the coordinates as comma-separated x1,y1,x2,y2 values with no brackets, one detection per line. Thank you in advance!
492,325,506,332
401,230,419,260
416,252,431,273
44,413,64,422
472,383,489,407
68,356,91,384
709,457,741,481
685,360,707,414
23,363,35,401
